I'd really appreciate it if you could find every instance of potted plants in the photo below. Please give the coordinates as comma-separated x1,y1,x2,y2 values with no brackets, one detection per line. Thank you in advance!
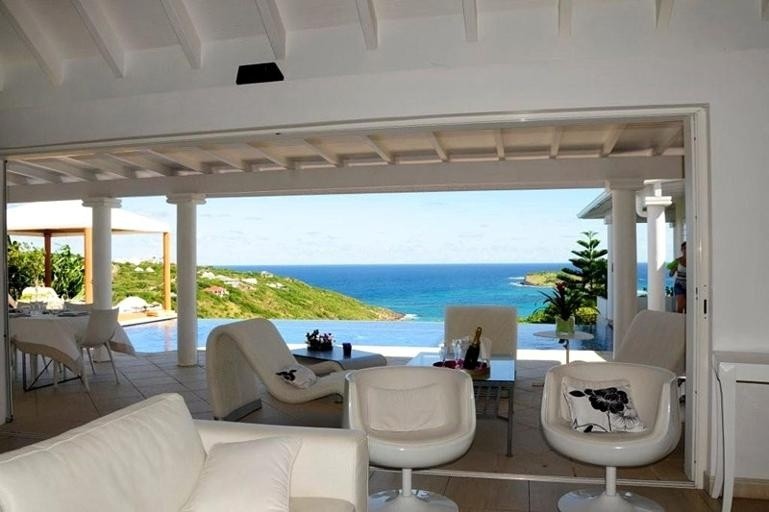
537,283,585,338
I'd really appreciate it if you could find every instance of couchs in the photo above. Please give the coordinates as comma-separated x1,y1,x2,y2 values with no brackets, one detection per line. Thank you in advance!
0,393,368,511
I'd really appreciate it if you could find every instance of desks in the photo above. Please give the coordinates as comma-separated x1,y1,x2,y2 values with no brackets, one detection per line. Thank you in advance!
7,311,135,392
712,351,769,512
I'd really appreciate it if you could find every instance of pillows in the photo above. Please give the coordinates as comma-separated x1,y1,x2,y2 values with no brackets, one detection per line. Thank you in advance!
179,437,303,512
561,375,648,432
276,364,317,389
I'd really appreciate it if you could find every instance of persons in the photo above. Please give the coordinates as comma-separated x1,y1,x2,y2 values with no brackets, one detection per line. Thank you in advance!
670,241,686,315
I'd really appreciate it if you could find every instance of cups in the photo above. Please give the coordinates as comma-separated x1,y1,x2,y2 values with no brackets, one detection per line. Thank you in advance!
440,346,448,367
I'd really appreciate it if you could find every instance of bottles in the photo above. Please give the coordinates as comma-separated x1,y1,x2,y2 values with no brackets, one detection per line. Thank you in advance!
463,327,482,370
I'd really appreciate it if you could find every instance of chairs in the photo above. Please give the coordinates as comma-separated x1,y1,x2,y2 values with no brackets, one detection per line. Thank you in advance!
206,317,347,421
444,307,516,361
541,363,683,512
62,302,96,312
613,308,685,378
54,307,119,391
342,365,476,512
